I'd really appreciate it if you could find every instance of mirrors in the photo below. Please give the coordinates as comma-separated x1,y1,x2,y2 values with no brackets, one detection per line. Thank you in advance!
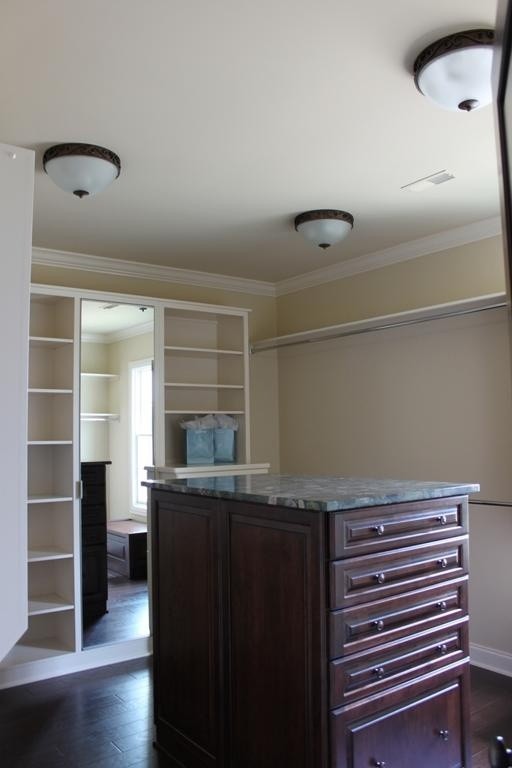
80,297,159,652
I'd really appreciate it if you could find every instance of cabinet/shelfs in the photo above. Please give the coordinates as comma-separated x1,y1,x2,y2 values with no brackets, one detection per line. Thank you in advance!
1,281,80,691
79,372,120,421
107,518,147,580
156,295,272,474
150,489,471,768
81,461,112,619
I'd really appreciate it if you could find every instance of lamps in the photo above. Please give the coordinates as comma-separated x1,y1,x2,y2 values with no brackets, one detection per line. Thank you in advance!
413,29,495,112
42,143,121,199
294,209,354,251
